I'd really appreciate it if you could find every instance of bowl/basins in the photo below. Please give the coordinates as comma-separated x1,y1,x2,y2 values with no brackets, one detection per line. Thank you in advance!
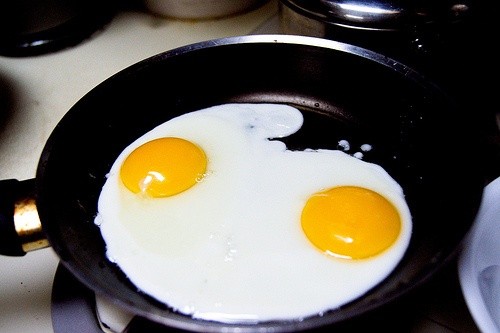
457,175,500,333
0,0,117,58
133,0,268,19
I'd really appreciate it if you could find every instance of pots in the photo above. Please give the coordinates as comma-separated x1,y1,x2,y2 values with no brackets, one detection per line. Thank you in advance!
278,0,475,73
0,33,485,333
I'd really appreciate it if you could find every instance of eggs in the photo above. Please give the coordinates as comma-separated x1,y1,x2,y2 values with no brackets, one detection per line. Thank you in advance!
94,104,412,324
